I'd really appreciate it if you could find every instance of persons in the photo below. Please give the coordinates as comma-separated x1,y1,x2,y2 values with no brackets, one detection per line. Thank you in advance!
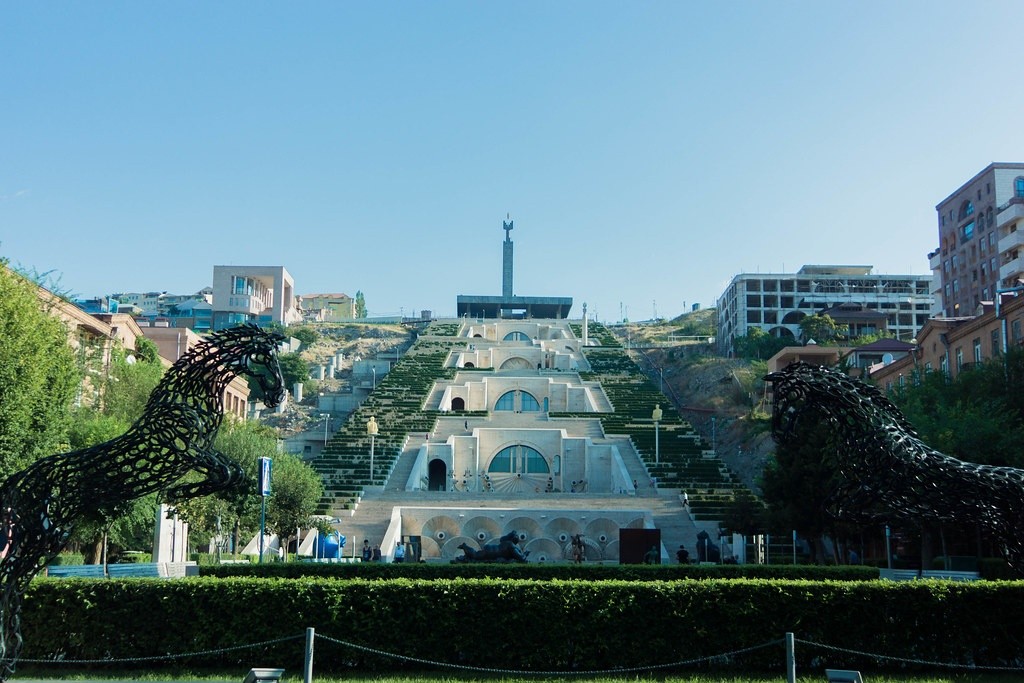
645,545,659,565
683,490,689,507
633,479,639,488
393,542,405,562
362,539,381,561
425,433,429,442
464,420,468,432
650,478,655,486
676,544,690,565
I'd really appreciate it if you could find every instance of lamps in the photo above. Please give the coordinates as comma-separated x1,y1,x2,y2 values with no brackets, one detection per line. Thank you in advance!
825,668,864,683
243,668,286,683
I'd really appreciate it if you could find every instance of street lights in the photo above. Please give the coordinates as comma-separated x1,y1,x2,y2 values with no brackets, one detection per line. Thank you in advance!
653,403,663,467
367,416,379,485
